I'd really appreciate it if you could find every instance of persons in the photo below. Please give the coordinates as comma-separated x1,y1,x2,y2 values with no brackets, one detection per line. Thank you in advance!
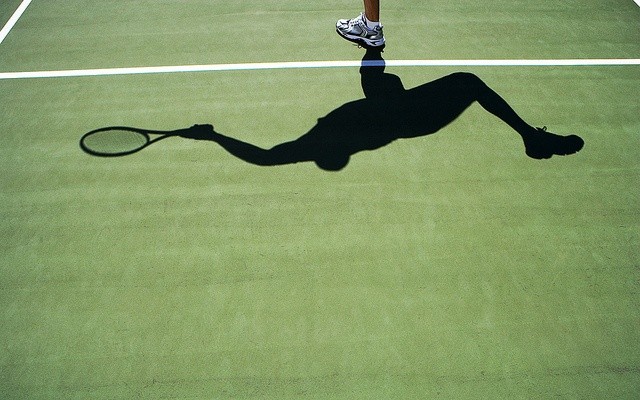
336,0,385,47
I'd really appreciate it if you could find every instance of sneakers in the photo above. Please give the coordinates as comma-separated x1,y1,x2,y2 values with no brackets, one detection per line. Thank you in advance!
336,13,386,47
